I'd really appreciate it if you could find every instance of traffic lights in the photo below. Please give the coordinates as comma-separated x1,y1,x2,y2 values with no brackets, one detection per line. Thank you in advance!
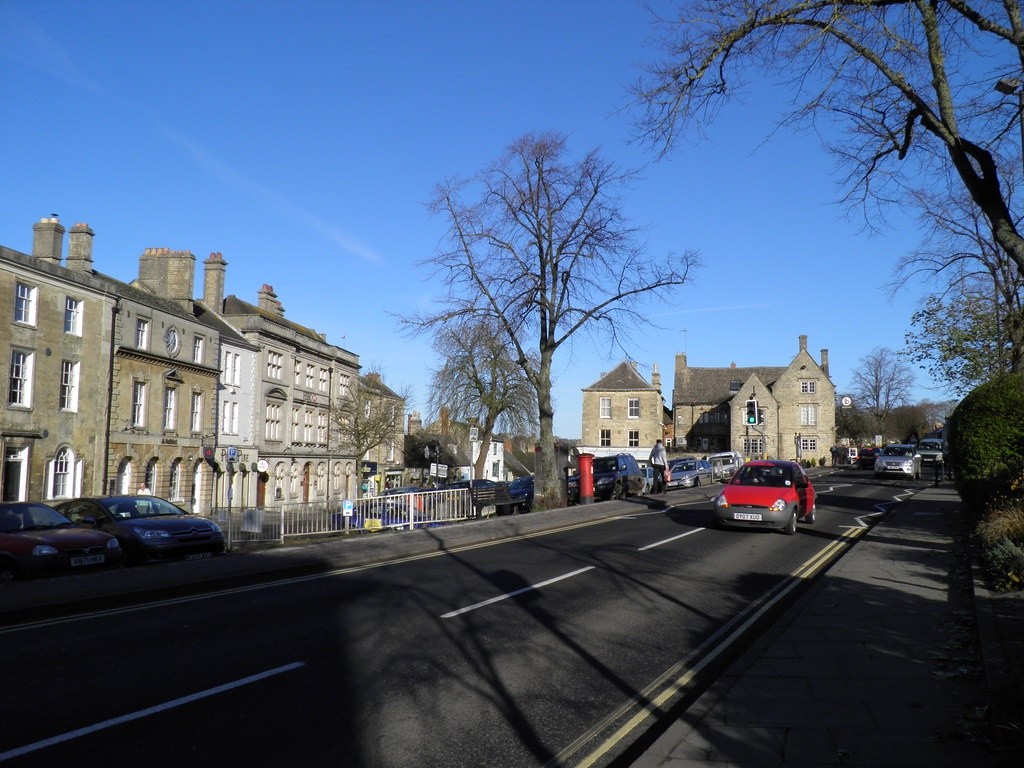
745,400,758,427
758,408,766,426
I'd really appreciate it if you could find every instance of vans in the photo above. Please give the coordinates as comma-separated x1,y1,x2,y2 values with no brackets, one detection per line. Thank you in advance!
918,438,948,467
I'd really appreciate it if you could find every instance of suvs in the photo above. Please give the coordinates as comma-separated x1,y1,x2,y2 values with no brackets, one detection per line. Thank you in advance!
874,444,923,481
566,452,645,502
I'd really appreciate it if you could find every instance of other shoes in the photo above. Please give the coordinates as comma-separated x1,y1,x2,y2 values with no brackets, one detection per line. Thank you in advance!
662,489,666,494
654,490,658,495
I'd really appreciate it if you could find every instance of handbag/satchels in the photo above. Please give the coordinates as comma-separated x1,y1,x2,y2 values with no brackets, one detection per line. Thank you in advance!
664,470,671,482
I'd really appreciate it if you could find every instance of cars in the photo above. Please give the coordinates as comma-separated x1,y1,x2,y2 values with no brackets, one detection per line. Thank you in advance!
48,494,226,559
666,458,713,490
639,467,662,496
705,451,744,478
334,502,443,534
857,448,881,468
0,501,124,582
374,475,537,516
714,459,818,536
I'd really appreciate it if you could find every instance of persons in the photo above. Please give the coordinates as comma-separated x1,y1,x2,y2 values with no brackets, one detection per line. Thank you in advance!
904,449,913,457
830,443,850,464
649,439,669,495
742,469,767,485
136,482,151,514
385,477,391,490
